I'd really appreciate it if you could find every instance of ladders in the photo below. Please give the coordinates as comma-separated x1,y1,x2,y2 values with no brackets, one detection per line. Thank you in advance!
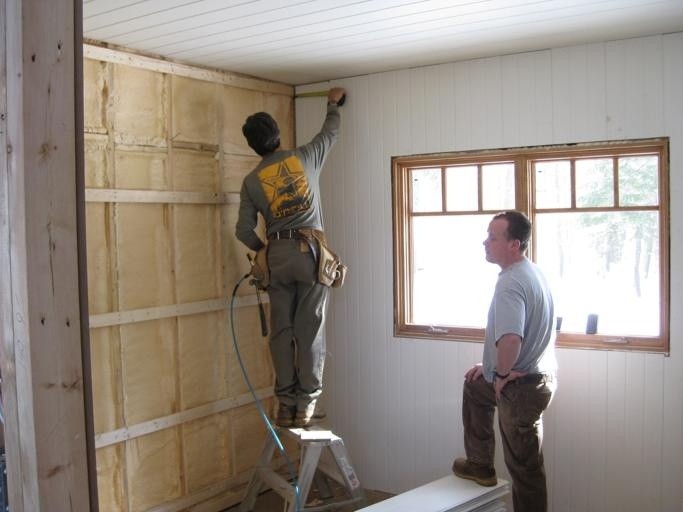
240,422,368,512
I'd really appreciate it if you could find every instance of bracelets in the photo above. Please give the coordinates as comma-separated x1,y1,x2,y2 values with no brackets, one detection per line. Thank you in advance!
495,373,510,380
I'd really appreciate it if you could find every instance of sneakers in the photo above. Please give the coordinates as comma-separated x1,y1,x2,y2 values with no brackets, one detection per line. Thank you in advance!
276,404,326,428
452,458,497,486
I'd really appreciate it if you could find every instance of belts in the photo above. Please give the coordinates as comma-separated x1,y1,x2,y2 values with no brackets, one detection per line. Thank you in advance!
509,373,555,384
267,230,308,240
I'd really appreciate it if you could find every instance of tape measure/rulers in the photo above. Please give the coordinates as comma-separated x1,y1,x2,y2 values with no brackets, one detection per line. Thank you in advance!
294,87,346,108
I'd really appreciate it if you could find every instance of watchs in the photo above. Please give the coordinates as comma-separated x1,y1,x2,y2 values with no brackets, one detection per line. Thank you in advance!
327,100,339,107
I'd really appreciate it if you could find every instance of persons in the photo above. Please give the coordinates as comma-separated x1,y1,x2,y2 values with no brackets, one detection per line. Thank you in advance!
234,87,346,428
451,210,557,512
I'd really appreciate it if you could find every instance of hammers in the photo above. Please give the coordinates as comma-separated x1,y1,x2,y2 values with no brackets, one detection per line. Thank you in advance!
249,279,268,336
